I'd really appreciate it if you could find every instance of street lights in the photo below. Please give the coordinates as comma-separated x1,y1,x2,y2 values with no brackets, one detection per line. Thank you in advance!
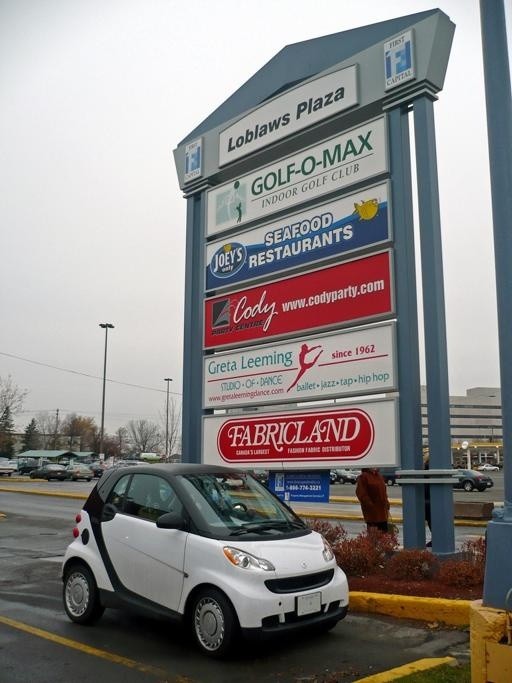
99,323,115,464
164,377,173,455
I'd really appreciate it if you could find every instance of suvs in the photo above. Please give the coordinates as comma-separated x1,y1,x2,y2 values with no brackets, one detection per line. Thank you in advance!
329,471,337,483
334,467,357,484
452,460,466,469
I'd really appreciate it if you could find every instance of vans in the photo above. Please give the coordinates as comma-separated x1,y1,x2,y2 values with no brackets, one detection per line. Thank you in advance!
376,466,401,485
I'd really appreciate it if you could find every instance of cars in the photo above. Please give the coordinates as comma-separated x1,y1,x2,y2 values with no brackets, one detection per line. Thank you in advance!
452,469,493,492
1,451,163,482
59,463,350,659
477,463,499,471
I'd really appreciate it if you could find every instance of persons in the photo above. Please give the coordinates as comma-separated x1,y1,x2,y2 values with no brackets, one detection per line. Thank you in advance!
424,459,432,548
355,468,390,533
